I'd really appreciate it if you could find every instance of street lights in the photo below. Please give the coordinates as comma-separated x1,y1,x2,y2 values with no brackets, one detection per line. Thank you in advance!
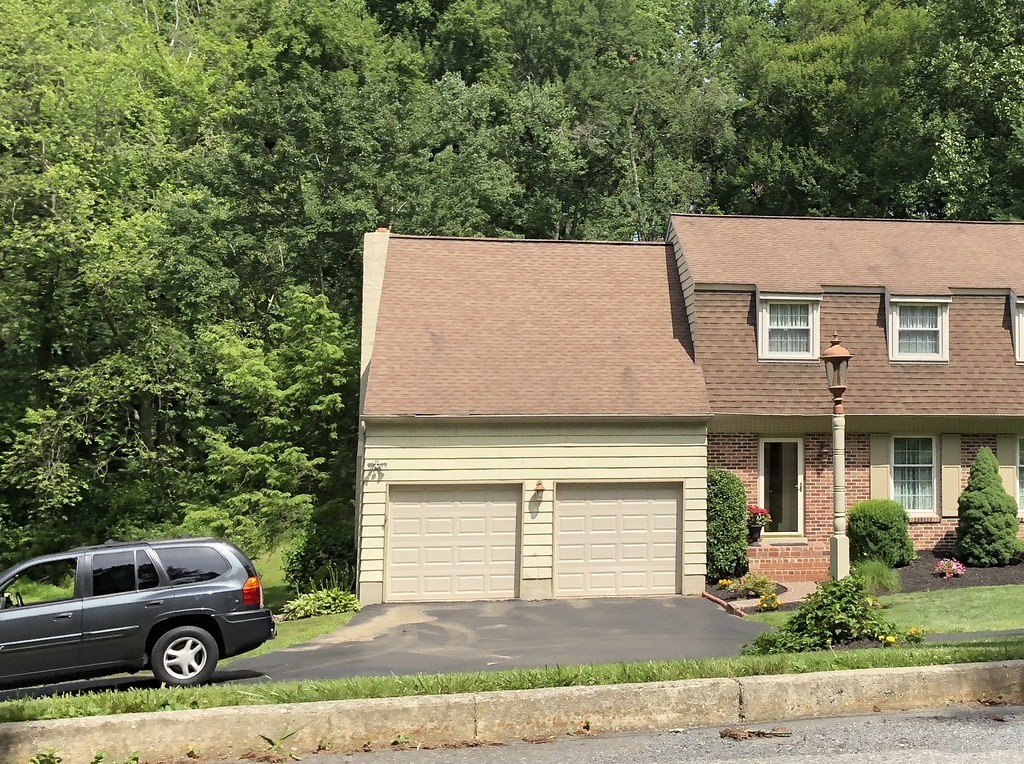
817,330,853,580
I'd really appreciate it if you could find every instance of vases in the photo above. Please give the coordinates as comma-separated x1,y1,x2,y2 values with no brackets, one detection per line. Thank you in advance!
746,524,763,540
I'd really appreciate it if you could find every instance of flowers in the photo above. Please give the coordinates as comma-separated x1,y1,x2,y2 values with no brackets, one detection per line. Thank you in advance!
746,505,772,526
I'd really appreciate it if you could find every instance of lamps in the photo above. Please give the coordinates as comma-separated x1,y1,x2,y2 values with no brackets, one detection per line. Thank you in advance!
533,481,545,500
820,442,830,464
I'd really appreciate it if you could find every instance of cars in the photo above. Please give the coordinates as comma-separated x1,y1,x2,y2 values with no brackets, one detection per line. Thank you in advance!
0,536,277,688
